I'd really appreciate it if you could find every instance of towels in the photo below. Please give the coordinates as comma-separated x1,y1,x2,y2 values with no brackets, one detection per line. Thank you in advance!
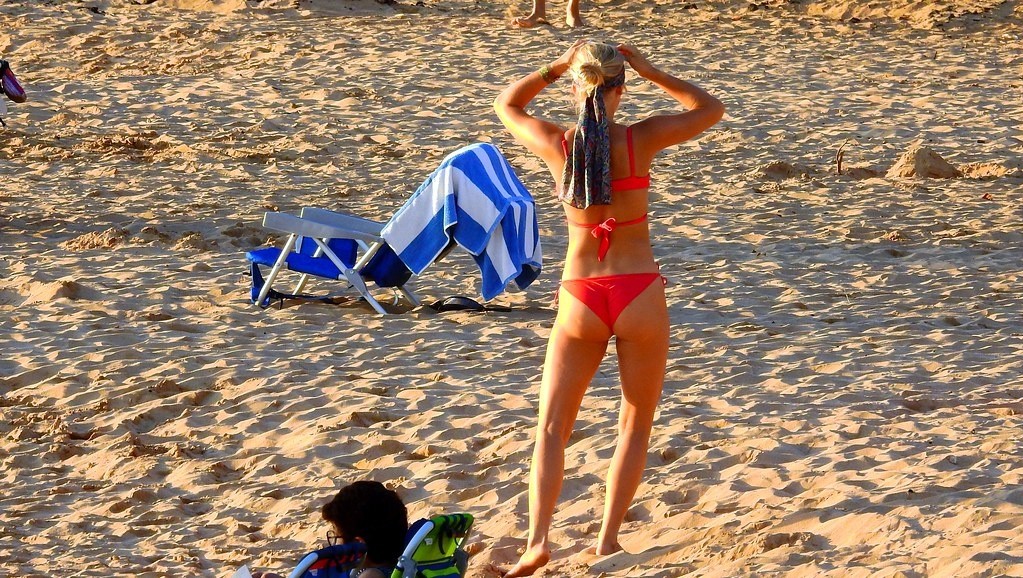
380,142,542,302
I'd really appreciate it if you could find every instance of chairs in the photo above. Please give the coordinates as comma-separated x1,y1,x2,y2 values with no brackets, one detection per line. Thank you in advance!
246,143,544,314
288,514,475,578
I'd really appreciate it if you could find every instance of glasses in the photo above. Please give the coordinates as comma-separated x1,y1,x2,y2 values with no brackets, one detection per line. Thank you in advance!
326,530,353,546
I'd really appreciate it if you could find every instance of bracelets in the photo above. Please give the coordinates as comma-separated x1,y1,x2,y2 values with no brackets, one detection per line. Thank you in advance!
540,64,560,84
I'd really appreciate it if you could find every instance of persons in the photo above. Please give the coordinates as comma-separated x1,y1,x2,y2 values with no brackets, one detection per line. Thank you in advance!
322,482,408,578
493,39,725,578
511,0,585,29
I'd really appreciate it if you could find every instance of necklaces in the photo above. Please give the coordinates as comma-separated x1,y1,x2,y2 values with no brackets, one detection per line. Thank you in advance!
355,568,366,577
